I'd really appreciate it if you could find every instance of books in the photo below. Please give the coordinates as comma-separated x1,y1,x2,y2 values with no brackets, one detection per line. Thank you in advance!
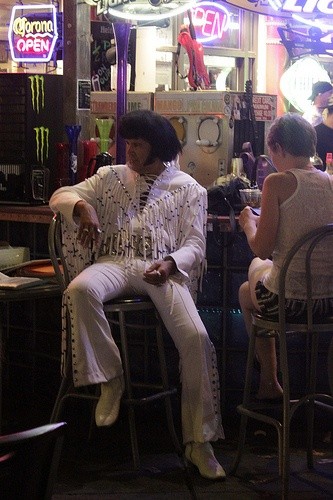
0,273,44,290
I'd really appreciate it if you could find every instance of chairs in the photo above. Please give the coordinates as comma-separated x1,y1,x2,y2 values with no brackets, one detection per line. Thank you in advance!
235,223,333,495
39,204,187,471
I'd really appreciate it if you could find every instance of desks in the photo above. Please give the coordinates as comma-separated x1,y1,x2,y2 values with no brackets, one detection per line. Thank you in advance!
0,258,70,419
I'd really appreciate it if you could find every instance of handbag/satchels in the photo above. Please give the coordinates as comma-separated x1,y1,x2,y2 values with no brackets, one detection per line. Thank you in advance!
207,177,250,248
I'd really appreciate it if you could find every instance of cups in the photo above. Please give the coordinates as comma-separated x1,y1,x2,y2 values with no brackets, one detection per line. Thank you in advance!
231,158,244,175
239,188,259,206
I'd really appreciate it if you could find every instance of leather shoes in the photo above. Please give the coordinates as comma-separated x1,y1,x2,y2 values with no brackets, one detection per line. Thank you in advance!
95,375,126,426
185,441,226,479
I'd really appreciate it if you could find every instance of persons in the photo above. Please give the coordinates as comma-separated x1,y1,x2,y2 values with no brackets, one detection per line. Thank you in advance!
238,113,333,407
302,81,333,128
48,110,226,480
312,105,333,172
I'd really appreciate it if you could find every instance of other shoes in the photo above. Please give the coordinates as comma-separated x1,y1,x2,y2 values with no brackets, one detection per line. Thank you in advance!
255,390,283,402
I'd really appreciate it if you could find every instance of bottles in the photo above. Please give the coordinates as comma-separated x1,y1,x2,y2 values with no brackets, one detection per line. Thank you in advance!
324,153,333,183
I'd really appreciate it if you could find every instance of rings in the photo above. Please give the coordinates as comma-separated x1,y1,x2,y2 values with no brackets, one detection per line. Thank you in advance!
156,271,161,277
96,229,102,234
83,229,88,233
